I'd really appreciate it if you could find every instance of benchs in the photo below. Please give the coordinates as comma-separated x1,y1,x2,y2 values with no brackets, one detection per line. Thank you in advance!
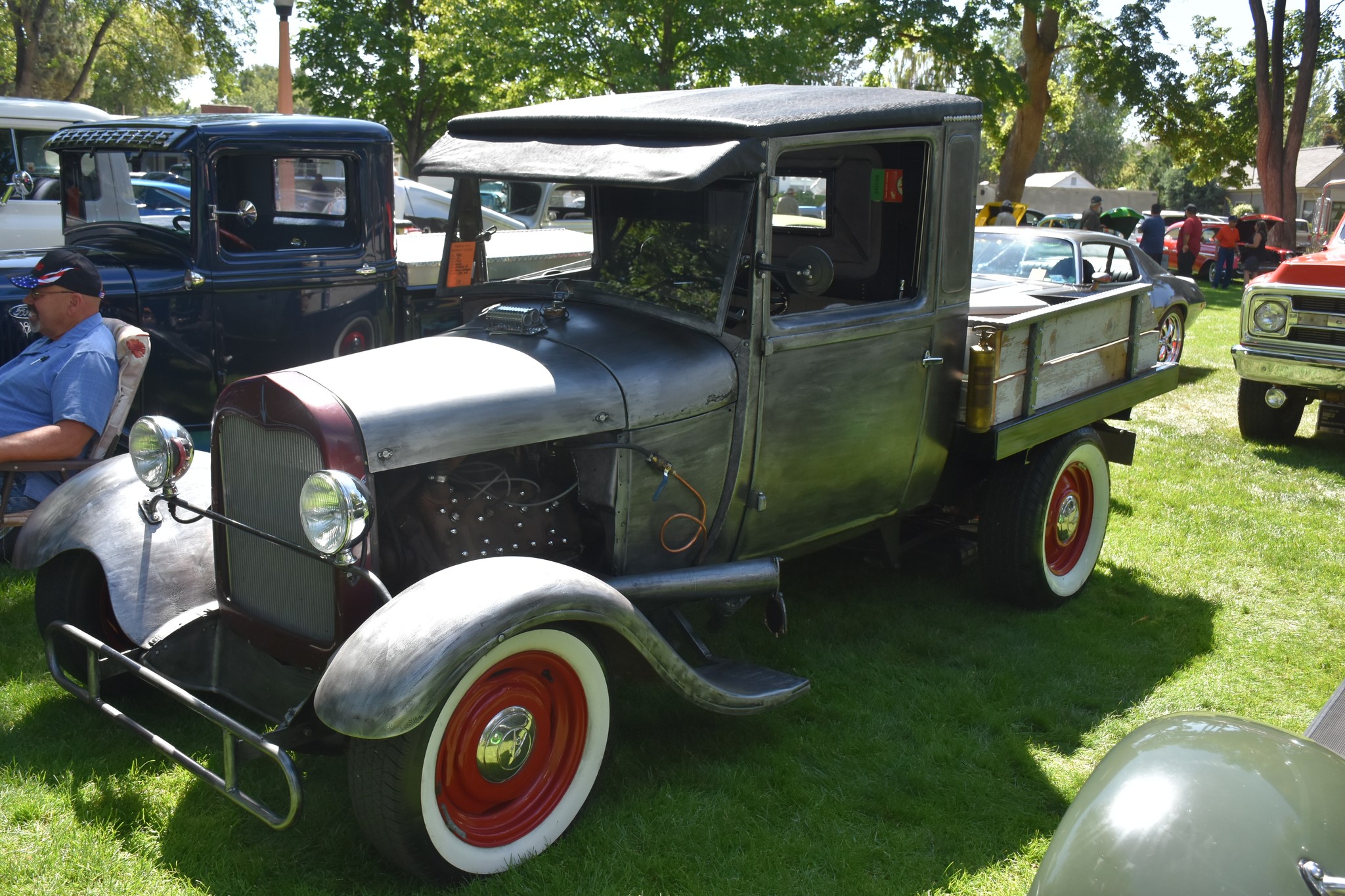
713,279,875,313
26,176,60,200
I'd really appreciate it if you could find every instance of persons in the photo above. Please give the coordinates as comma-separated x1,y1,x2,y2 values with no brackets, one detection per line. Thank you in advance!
1243,221,1267,291
993,199,1016,227
1177,204,1203,279
1211,215,1241,290
0,244,123,518
1137,204,1165,267
308,173,327,214
1080,196,1107,231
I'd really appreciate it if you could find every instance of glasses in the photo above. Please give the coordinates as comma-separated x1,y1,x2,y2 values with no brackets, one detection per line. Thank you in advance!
29,289,75,299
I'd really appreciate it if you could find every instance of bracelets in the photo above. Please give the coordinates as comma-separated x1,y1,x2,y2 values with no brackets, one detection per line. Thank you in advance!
1244,243,1248,247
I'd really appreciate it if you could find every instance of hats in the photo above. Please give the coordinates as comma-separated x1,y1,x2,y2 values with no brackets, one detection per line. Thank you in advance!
1184,203,1197,212
5,250,105,298
1091,196,1101,203
1228,215,1239,221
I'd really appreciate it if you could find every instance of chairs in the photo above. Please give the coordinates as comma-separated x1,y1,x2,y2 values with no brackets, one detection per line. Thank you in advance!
0,316,151,538
1049,258,1095,284
